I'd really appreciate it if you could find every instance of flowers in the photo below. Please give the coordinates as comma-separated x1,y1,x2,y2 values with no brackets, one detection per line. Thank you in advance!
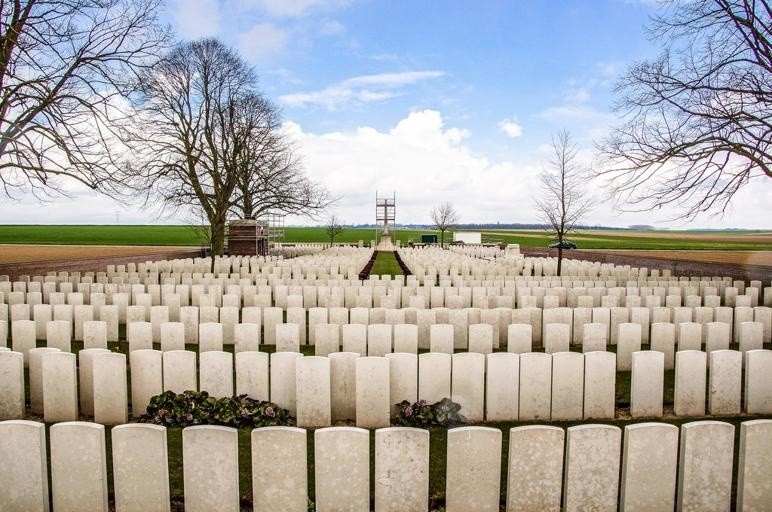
146,389,290,430
393,395,459,429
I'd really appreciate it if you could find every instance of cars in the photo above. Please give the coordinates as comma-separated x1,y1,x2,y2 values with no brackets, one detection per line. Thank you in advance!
549,241,577,250
